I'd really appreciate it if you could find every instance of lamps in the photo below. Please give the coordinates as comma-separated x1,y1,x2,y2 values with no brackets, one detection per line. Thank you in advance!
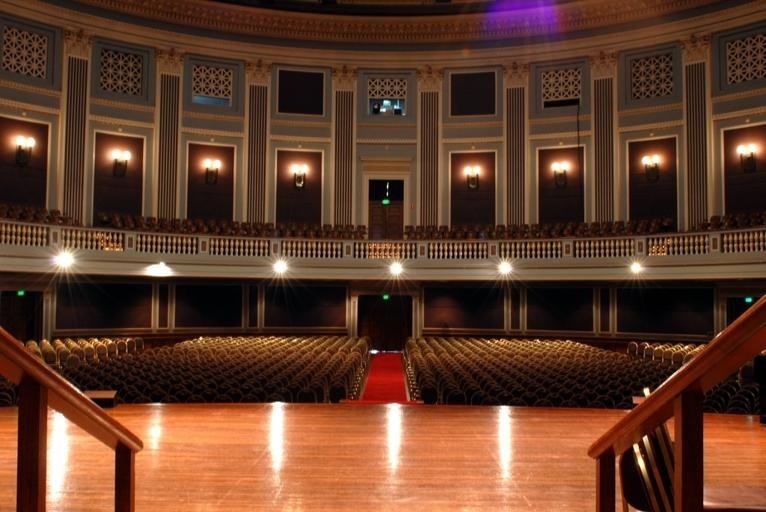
460,140,764,190
8,133,313,191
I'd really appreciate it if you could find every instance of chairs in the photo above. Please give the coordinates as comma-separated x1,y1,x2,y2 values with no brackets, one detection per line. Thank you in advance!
0,205,366,239
0,336,369,414
402,336,765,415
405,211,766,244
